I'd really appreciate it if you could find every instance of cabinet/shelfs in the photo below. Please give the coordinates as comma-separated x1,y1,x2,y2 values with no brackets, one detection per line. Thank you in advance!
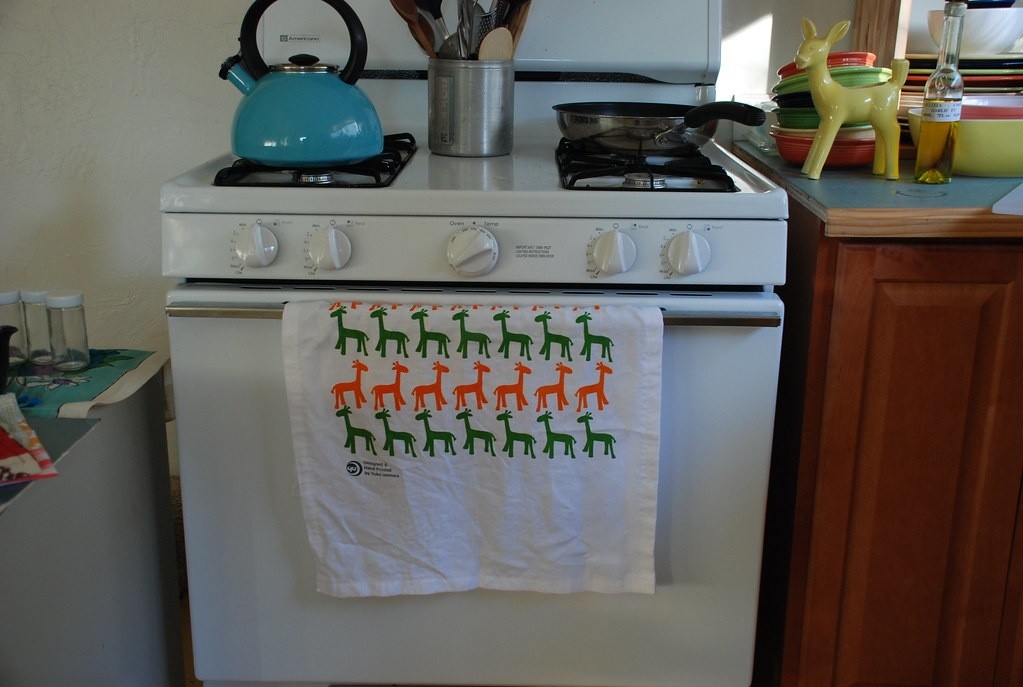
853,0,1023,118
731,133,1023,687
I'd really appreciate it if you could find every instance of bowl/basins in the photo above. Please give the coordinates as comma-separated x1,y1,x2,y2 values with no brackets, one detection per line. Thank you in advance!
927,7,1022,56
908,96,1023,179
770,52,891,167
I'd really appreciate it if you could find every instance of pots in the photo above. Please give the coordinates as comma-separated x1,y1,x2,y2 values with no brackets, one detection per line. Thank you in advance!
552,101,766,157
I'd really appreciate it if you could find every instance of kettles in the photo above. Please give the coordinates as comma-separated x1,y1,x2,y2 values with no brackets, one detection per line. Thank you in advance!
218,0,384,169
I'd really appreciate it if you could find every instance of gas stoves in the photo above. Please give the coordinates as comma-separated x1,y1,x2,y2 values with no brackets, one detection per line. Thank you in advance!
159,0,789,285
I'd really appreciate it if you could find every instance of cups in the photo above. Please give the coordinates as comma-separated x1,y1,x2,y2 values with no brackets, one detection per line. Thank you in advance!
427,58,514,157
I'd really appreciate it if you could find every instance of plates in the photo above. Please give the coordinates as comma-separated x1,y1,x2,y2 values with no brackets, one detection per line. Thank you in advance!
897,54,1023,140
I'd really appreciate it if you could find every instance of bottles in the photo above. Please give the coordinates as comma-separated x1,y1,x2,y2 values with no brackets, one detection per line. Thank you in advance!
21,285,65,366
912,3,966,185
0,289,29,365
45,289,90,371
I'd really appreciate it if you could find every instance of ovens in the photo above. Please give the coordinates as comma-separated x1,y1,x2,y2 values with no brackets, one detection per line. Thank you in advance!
167,278,785,687
